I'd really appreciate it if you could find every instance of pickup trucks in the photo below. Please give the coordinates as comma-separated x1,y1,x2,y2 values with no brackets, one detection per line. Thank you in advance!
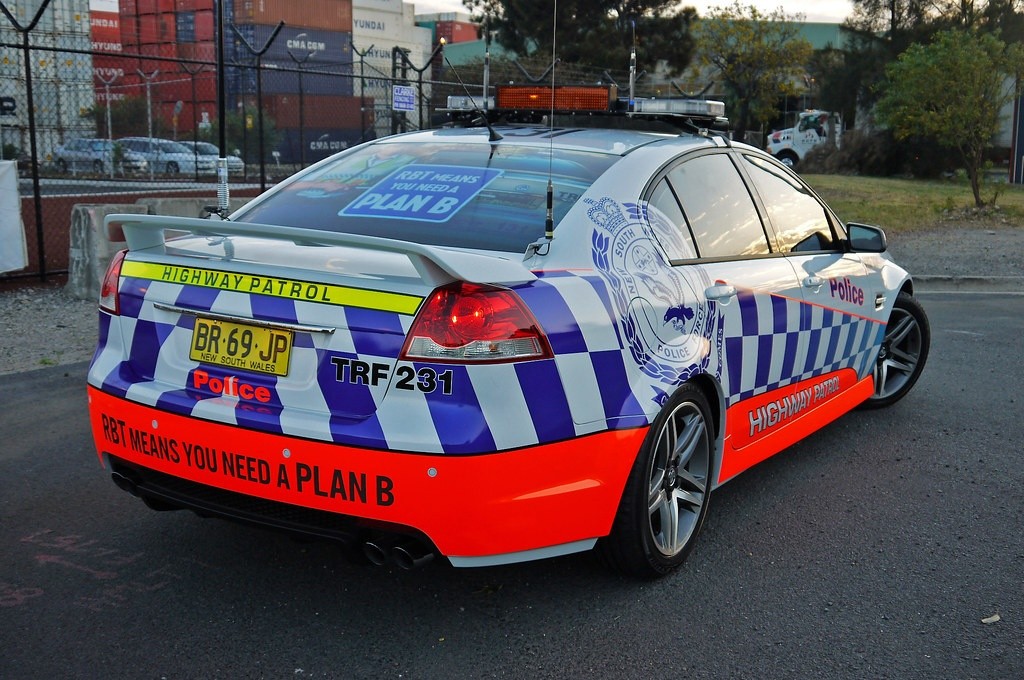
766,109,860,169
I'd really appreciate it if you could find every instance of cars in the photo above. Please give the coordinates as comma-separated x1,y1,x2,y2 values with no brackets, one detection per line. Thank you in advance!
53,138,147,176
178,139,245,174
112,136,218,177
86,3,937,578
